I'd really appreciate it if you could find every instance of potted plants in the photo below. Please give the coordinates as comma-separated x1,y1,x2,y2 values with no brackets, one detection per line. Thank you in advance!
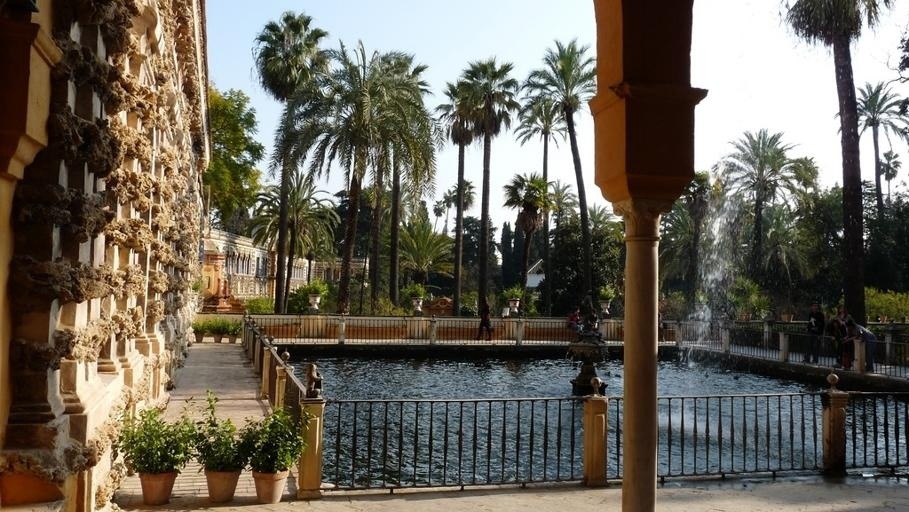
116,398,317,505
502,282,524,314
191,316,242,343
300,277,329,311
597,285,616,314
400,283,427,314
725,276,773,321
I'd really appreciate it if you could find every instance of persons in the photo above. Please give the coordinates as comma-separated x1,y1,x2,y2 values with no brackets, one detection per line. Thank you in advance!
474,295,493,340
582,308,604,343
566,305,585,341
802,302,878,373
